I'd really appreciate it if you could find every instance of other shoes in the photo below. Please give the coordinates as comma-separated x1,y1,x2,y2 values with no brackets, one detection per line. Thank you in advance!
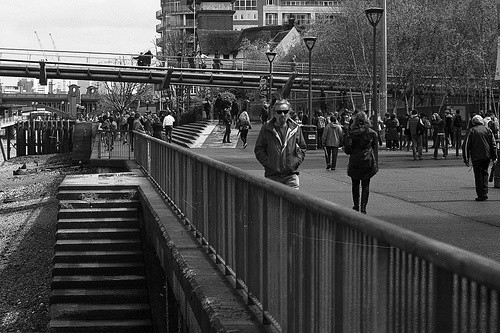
332,168,335,170
326,164,331,169
475,194,488,201
243,142,247,148
227,141,231,143
223,140,227,143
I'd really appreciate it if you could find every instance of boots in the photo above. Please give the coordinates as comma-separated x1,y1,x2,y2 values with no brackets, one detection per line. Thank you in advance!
353,205,359,211
361,203,366,214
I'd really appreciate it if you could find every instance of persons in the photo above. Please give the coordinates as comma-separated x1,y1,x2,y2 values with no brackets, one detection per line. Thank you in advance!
176,51,223,74
291,55,298,73
311,108,358,149
96,111,129,151
444,109,500,188
127,112,162,152
321,115,343,170
216,93,250,125
343,110,379,215
369,111,403,150
462,115,497,201
76,112,96,124
403,109,453,161
223,105,232,143
163,113,175,143
289,108,310,127
133,50,153,71
236,111,253,149
254,99,307,191
259,101,269,126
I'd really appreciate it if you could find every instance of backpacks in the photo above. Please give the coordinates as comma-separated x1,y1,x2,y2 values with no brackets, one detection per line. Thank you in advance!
317,116,325,129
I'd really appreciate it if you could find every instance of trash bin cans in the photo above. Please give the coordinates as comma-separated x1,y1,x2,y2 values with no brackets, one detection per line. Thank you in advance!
300,125,318,150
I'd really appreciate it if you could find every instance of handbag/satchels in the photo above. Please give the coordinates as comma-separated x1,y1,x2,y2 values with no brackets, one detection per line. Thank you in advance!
417,118,426,135
397,126,401,132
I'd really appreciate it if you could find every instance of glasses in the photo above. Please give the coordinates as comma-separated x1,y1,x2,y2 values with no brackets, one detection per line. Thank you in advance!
275,110,289,114
